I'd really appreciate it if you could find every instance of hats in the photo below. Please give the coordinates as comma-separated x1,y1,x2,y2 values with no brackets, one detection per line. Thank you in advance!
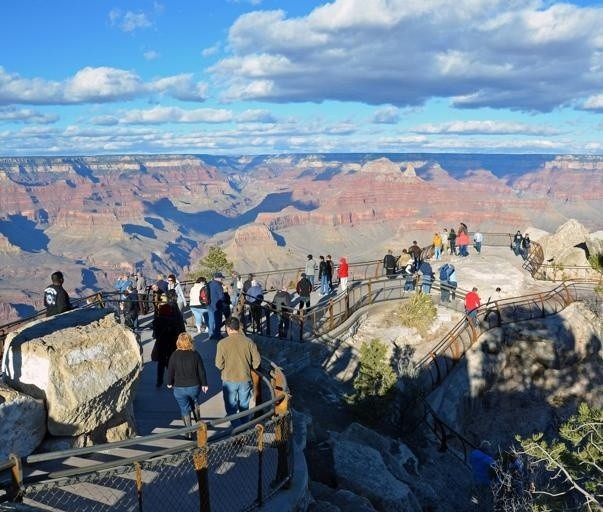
215,272,225,278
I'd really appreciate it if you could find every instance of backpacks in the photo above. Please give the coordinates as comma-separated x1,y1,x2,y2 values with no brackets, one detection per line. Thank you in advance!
199,281,211,306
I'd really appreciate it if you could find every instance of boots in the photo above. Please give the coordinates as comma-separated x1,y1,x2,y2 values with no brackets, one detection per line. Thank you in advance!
193,408,201,423
182,415,192,440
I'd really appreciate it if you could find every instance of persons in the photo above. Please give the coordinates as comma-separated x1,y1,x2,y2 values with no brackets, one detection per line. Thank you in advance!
297,273,313,321
150,272,264,339
272,286,292,339
43,271,78,317
484,287,504,322
507,453,525,497
304,255,349,296
470,440,497,512
464,287,481,328
514,230,522,256
383,223,482,303
152,289,186,387
215,317,261,434
166,332,208,441
116,271,146,335
522,233,530,261
